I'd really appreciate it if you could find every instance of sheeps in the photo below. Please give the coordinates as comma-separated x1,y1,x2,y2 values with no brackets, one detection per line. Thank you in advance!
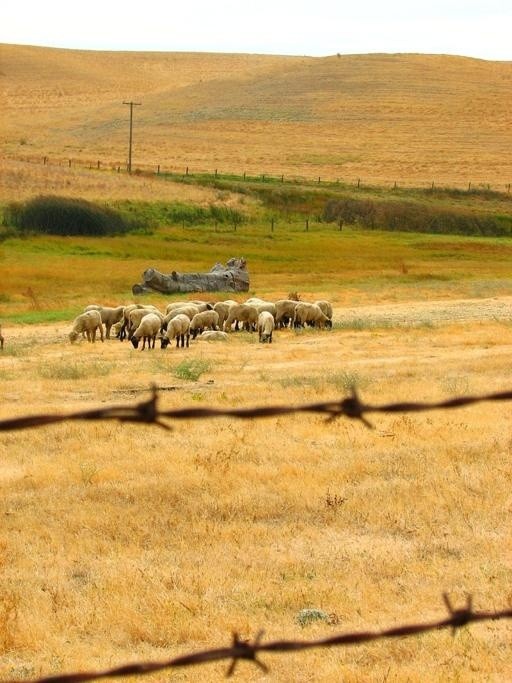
69,309,105,346
84,302,126,339
116,297,333,351
